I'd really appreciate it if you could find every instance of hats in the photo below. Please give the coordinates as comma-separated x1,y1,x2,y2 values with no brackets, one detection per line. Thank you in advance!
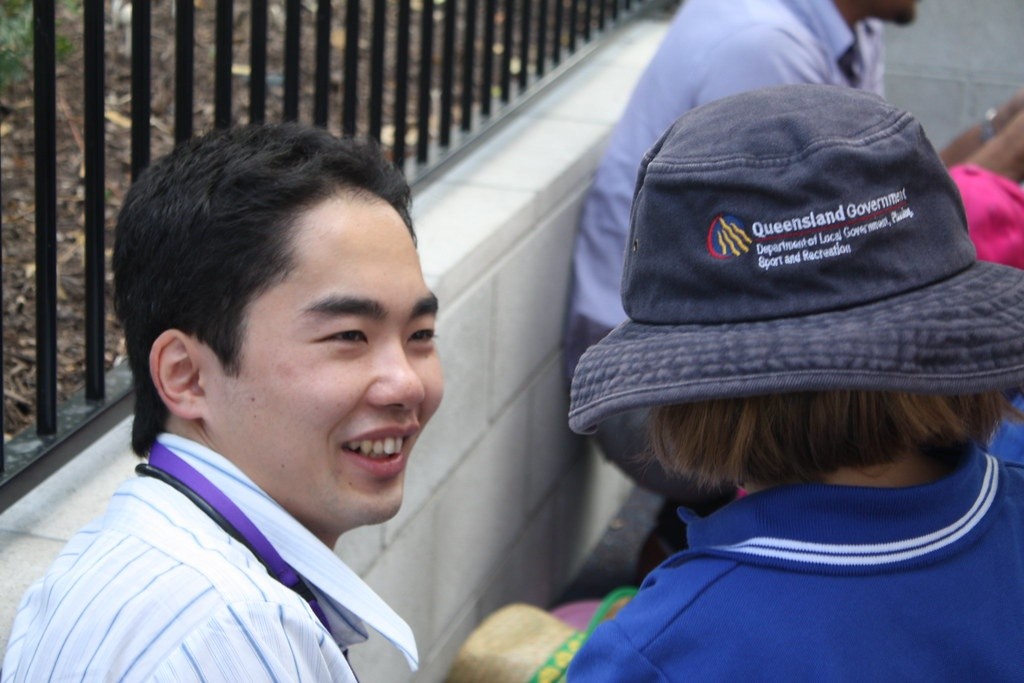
947,162,1024,269
441,585,639,683
567,82,1024,435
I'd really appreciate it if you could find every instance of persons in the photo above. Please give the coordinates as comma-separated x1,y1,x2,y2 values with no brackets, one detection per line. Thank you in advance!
0,123,445,683
562,0,1024,683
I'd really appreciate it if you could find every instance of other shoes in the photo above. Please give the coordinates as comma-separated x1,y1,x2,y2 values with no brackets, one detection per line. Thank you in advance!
636,526,691,588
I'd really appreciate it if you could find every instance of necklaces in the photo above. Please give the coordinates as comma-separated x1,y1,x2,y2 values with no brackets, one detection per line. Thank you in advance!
135,437,333,637
